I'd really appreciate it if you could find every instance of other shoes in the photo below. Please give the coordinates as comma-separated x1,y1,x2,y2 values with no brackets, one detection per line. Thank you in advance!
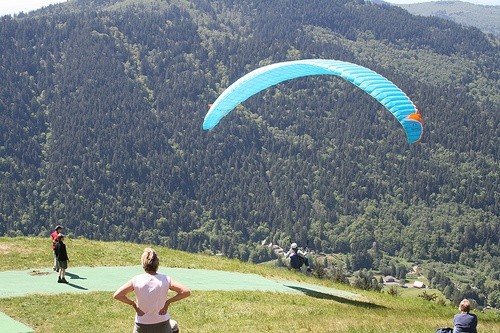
53,267,59,272
58,277,68,283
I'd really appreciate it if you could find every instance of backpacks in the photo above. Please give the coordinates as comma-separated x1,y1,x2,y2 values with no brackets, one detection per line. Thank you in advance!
291,254,302,268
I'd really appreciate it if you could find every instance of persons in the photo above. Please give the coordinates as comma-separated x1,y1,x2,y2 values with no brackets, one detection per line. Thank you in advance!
55,233,70,283
453,299,477,333
286,243,313,272
50,225,63,272
113,247,190,333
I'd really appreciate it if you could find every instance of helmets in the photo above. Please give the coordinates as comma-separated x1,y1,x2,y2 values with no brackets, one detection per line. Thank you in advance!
291,243,297,248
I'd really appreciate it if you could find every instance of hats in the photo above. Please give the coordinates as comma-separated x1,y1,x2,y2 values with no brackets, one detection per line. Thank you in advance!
55,225,64,231
